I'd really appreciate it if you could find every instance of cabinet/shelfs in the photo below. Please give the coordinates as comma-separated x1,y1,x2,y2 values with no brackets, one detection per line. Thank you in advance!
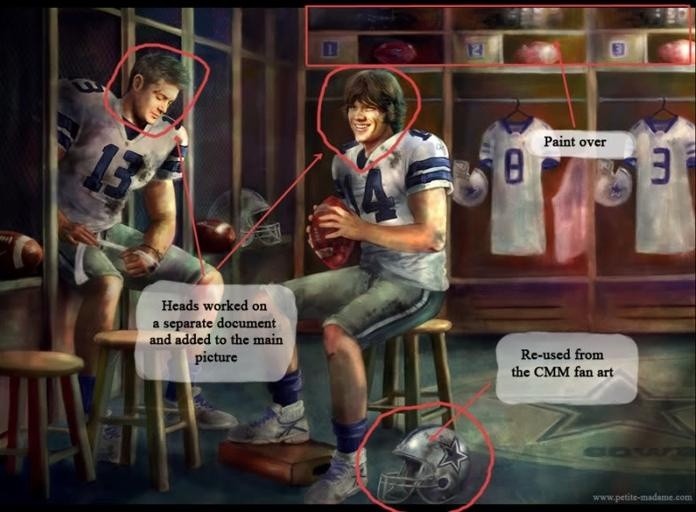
2,4,279,296
292,7,696,343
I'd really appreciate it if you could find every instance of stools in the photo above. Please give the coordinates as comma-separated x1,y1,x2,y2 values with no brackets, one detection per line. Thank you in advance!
18,345,98,500
87,324,209,491
364,315,461,441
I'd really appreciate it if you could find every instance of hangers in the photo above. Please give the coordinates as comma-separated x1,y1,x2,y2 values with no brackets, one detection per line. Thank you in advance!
645,95,673,127
500,97,532,126
